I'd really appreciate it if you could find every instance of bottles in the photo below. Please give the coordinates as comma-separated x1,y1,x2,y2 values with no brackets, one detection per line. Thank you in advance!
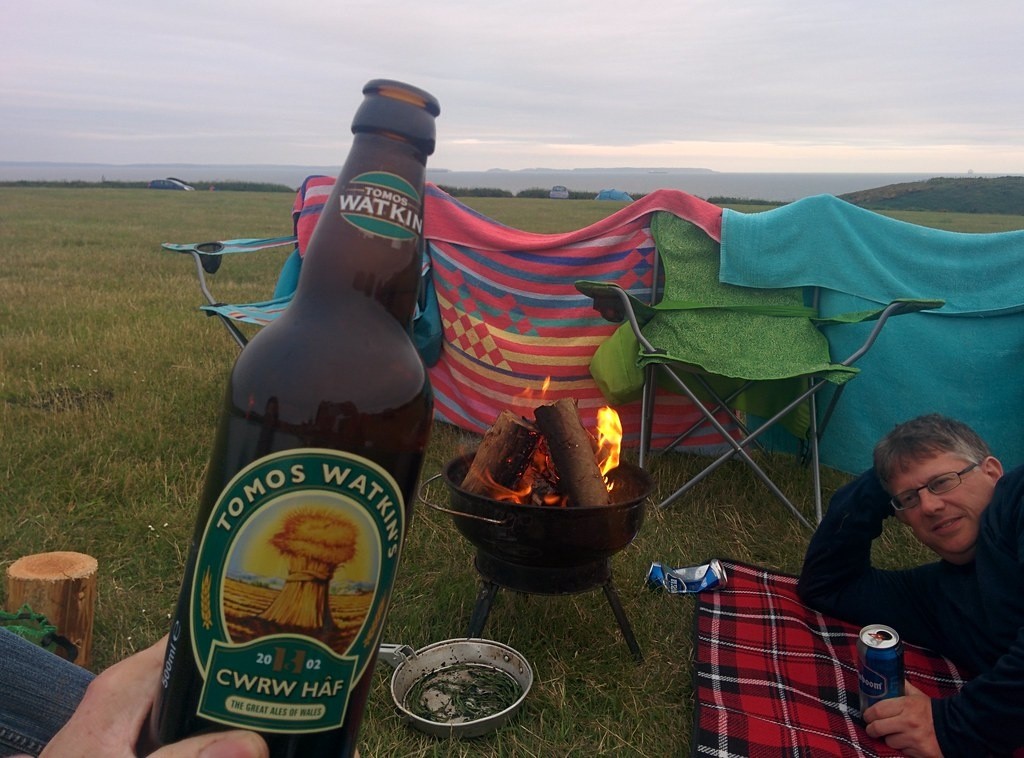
136,73,442,757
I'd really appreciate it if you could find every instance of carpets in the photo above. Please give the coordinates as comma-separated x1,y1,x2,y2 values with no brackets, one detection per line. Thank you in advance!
693,553,966,758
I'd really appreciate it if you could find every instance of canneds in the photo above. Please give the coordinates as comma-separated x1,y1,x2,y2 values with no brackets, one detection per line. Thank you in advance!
643,560,726,596
854,626,906,725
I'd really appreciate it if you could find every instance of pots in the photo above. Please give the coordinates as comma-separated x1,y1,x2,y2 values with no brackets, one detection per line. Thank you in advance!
378,638,535,737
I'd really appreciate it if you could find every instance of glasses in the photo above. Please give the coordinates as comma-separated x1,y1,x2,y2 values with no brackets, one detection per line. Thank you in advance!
888,463,979,512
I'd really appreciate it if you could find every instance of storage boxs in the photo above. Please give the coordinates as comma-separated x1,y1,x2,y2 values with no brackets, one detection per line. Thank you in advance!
391,637,533,741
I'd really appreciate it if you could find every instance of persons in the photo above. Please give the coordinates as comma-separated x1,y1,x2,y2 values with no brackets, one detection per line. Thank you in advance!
0,625,268,758
795,414,1024,758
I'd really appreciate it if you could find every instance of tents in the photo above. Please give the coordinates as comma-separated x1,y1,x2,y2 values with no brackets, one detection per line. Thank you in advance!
594,189,632,201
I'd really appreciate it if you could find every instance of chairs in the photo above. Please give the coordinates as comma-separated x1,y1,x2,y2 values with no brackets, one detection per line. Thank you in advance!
573,196,946,549
160,178,448,371
